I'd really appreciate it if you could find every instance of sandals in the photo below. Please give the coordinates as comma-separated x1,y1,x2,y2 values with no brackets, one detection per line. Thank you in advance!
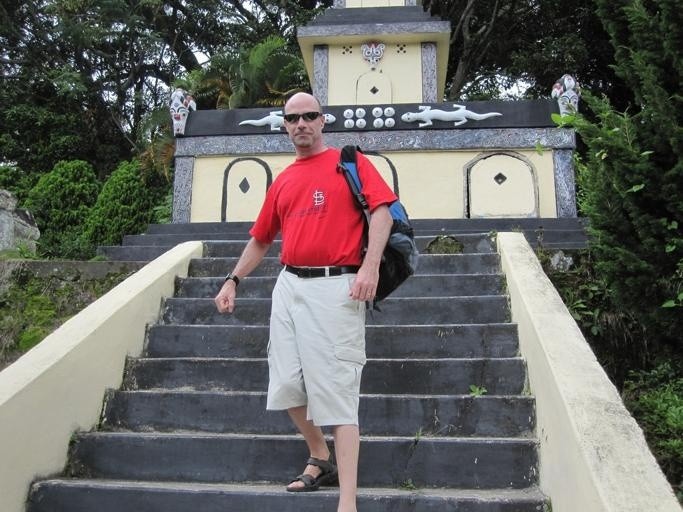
286,454,338,492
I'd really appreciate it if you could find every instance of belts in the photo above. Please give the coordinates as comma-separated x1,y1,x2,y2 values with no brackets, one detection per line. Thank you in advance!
286,265,360,278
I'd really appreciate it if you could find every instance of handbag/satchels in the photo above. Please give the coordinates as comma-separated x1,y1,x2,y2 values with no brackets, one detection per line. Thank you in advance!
361,201,419,308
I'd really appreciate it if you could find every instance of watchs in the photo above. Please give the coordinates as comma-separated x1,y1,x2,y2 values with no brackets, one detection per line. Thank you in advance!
224,272,240,287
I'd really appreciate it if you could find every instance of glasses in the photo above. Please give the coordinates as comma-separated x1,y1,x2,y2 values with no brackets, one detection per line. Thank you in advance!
284,112,322,124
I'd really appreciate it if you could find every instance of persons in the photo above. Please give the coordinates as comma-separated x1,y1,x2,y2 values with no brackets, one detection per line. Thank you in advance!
215,92,398,512
